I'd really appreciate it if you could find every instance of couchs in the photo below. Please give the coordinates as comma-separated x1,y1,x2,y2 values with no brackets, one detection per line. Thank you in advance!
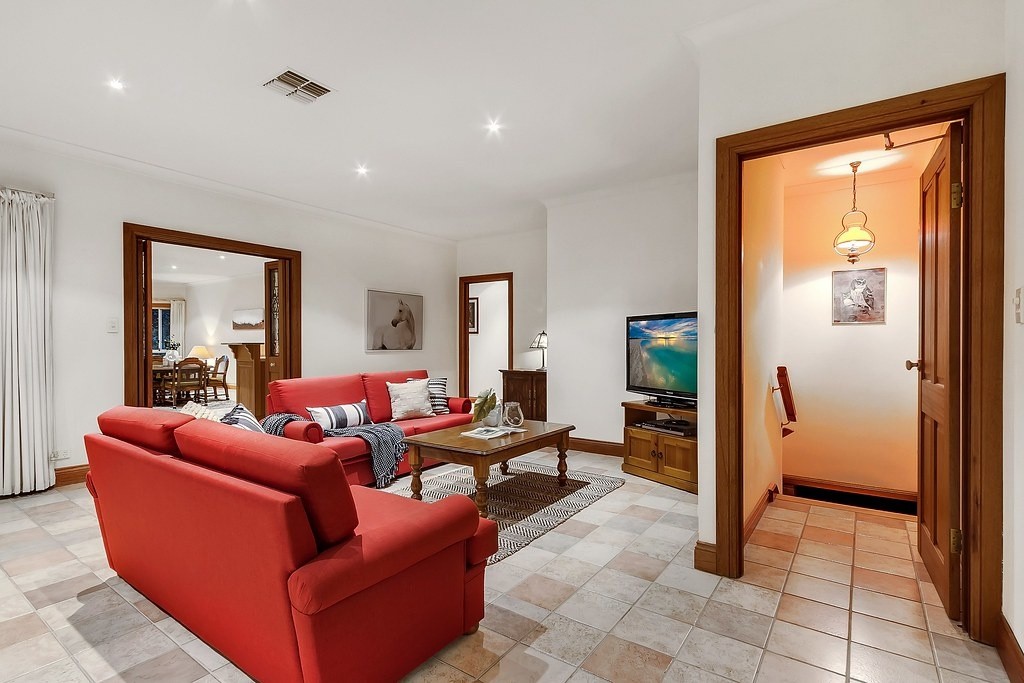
83,403,500,683
258,369,475,486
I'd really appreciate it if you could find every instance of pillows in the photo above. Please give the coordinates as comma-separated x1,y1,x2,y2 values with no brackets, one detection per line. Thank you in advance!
180,401,222,424
406,377,451,415
305,398,374,434
221,402,267,434
385,378,437,422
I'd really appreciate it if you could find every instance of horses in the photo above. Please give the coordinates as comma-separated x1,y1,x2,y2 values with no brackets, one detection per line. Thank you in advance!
371,297,416,350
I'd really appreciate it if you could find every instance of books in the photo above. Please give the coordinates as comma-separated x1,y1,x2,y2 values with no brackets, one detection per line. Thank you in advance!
461,425,528,439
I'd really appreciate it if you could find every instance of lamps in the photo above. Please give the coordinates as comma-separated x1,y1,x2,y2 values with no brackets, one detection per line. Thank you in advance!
187,345,212,361
529,331,547,372
834,160,876,266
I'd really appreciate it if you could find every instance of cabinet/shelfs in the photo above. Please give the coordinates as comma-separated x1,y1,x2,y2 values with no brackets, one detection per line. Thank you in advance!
222,342,266,421
621,399,699,495
498,370,547,422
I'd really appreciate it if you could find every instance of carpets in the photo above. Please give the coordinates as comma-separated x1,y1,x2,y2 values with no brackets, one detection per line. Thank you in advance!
389,459,625,568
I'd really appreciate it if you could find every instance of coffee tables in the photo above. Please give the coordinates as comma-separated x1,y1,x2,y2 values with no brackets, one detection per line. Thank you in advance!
400,417,576,518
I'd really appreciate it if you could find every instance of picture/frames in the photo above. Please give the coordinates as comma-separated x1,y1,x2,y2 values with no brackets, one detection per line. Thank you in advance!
831,267,887,326
363,287,425,353
468,297,480,335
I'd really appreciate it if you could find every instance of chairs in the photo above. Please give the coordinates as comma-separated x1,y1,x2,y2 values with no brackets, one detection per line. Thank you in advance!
152,355,229,409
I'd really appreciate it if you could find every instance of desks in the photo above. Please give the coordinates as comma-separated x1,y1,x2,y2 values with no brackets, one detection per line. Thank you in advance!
152,365,212,404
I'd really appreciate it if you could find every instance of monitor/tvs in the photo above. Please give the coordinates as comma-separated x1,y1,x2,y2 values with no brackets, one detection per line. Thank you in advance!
625,310,697,410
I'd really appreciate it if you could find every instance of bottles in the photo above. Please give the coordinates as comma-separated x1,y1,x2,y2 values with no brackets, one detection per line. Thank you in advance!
503,402,524,427
